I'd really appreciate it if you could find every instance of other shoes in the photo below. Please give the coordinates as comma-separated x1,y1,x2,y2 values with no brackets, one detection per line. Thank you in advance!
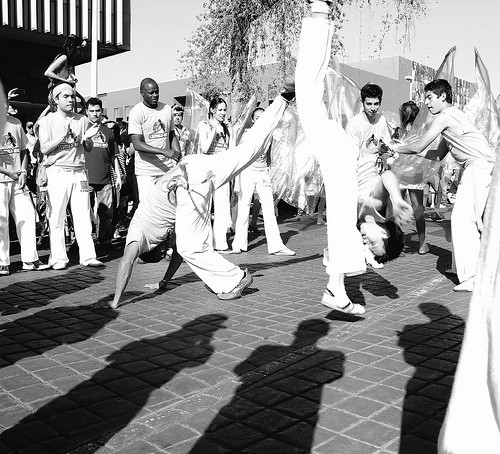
430,205,435,208
100,242,117,251
419,244,431,255
215,248,234,254
454,277,475,292
445,269,456,274
217,268,253,300
284,75,295,93
439,203,446,208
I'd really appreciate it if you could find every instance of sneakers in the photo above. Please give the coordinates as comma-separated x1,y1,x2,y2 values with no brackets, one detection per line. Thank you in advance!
320,288,365,315
274,246,296,256
307,0,332,5
22,261,50,270
232,244,242,256
0,265,9,274
87,260,104,267
367,256,385,269
453,277,475,292
52,261,66,269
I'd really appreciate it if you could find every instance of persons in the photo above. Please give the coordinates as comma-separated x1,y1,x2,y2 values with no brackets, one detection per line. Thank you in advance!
0,35,500,292
90,81,297,309
294,0,416,315
438,144,500,454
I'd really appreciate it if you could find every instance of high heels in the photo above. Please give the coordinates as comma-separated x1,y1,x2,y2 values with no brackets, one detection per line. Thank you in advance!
249,224,260,233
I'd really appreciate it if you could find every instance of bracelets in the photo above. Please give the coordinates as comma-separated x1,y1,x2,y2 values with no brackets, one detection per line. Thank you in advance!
21,169,27,176
162,280,168,282
